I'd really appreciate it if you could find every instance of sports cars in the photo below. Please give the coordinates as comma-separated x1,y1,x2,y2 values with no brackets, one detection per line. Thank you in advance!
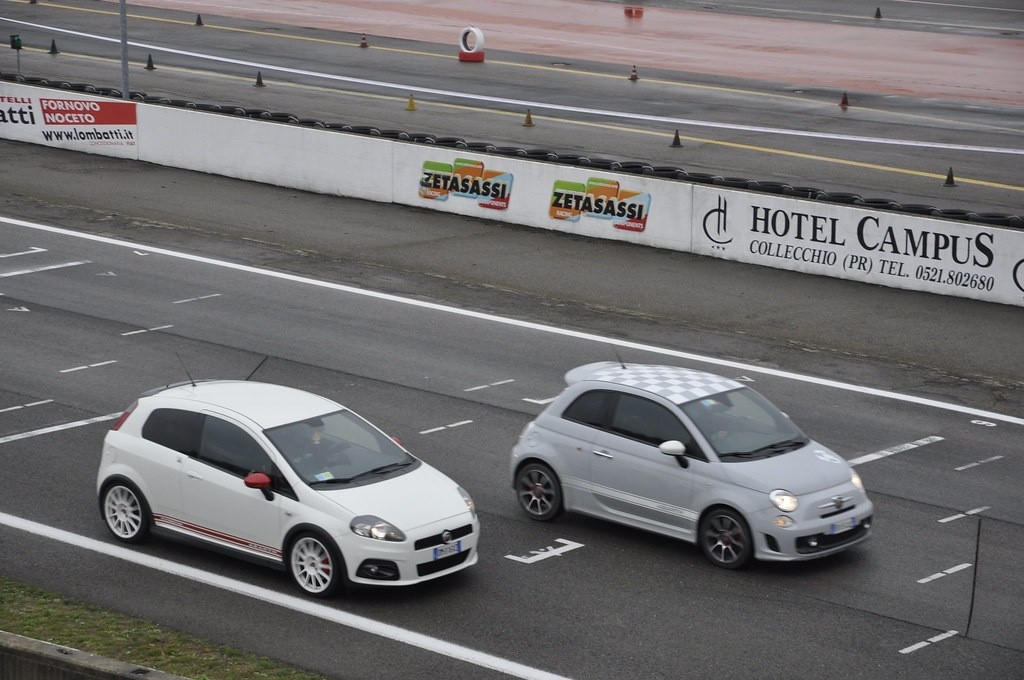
509,359,875,571
96,376,481,600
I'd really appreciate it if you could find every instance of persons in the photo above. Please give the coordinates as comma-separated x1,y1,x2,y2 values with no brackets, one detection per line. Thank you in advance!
303,419,352,473
673,403,729,444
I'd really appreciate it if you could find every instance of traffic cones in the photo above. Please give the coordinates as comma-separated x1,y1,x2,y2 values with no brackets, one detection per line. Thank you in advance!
252,70,267,87
27,0,39,3
195,12,204,26
874,4,884,20
669,129,685,148
522,107,536,126
143,53,157,69
942,166,959,188
629,64,642,79
838,92,852,107
48,39,60,55
405,91,423,111
358,32,370,48
